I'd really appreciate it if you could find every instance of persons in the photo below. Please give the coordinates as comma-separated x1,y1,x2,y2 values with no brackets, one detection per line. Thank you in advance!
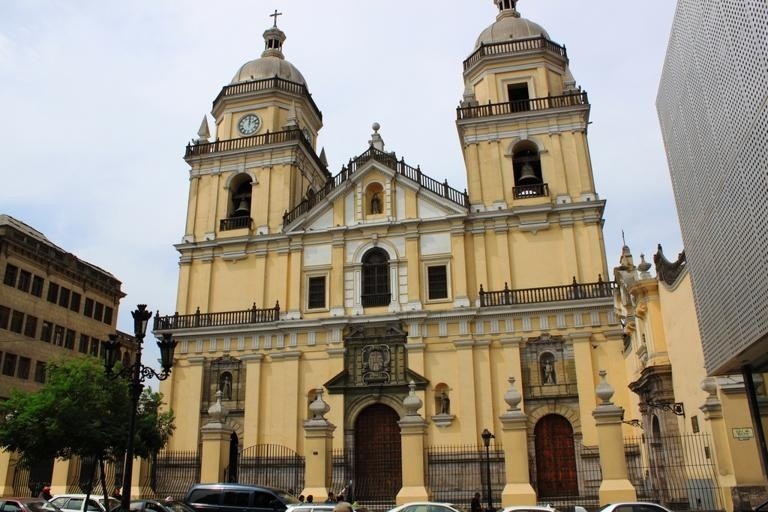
38,487,53,501
112,487,121,500
471,492,481,512
222,375,231,399
288,489,294,496
544,360,553,383
299,492,344,503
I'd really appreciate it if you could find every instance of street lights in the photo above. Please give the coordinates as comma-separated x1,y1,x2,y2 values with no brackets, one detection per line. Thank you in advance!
102,301,180,511
480,426,496,511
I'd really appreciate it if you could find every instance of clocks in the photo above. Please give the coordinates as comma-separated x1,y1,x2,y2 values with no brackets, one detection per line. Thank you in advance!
303,125,312,146
237,112,262,138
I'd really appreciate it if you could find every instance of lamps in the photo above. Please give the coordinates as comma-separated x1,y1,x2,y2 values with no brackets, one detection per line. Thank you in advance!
619,406,638,427
641,386,684,415
